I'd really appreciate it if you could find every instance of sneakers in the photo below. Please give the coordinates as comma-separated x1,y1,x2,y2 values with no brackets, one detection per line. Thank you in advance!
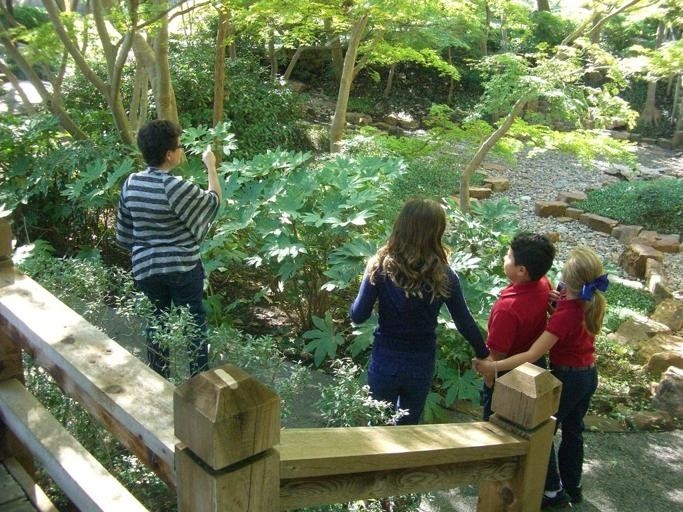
542,483,585,512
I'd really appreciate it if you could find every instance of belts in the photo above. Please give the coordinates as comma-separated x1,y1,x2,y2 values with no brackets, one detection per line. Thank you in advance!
555,362,597,372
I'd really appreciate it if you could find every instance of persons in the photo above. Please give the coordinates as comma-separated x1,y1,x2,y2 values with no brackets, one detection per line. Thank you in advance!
351,199,492,431
469,246,610,512
113,118,221,379
479,231,574,512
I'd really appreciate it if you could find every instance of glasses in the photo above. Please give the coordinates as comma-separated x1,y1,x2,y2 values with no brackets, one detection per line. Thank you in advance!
176,143,185,148
